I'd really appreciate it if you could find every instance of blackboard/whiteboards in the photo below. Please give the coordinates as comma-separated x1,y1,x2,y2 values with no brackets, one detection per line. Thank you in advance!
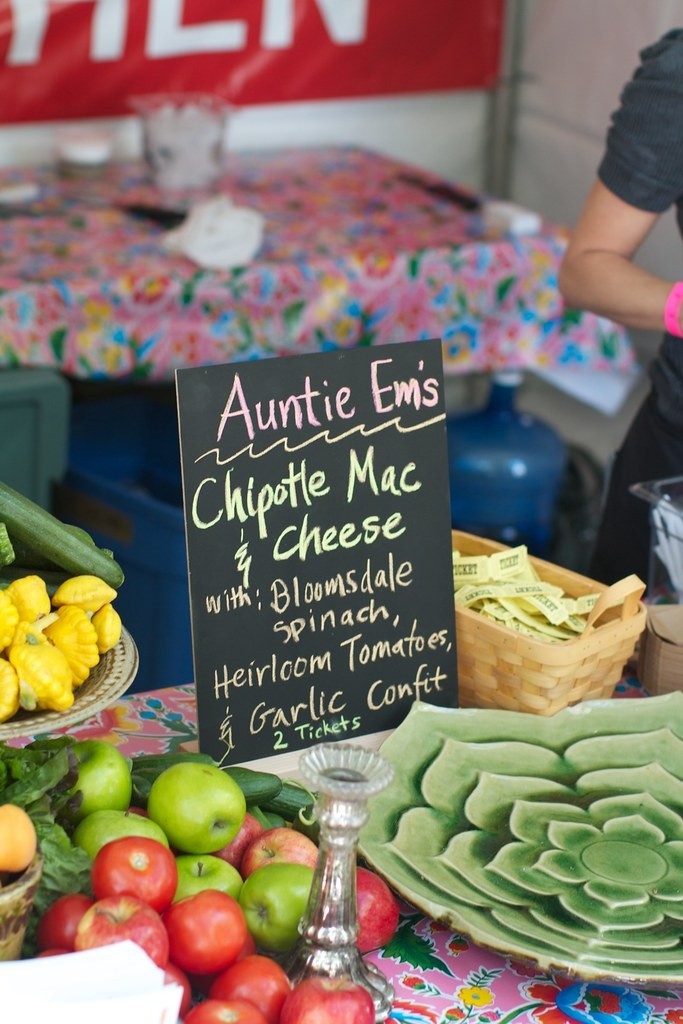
172,337,459,766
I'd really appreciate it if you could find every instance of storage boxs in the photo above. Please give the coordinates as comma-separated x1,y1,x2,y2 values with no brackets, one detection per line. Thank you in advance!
629,480,682,606
638,596,682,695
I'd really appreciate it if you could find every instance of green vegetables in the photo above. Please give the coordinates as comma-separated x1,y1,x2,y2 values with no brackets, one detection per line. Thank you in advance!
0,736,98,961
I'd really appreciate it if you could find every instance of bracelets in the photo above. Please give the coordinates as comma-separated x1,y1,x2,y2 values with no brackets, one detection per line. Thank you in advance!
664,280,683,338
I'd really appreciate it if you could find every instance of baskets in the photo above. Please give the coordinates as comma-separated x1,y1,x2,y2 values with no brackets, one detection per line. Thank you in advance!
451,528,648,717
0,626,139,741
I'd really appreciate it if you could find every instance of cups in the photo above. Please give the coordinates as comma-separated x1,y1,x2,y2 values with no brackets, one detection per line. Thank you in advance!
55,137,113,184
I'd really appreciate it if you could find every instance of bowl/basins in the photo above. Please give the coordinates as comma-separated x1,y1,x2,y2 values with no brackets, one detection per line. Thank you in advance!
627,474,683,646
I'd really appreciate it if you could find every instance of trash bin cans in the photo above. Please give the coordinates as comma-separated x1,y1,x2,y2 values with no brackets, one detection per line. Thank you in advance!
51,388,193,695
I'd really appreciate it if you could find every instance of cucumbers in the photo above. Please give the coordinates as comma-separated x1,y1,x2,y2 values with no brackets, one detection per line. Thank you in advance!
126,751,321,836
0,479,127,605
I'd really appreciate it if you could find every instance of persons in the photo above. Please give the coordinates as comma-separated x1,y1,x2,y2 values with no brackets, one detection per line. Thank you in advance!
559,29,683,595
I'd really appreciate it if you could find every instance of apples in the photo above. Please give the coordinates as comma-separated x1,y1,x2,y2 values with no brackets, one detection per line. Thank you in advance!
33,762,399,1024
57,741,133,824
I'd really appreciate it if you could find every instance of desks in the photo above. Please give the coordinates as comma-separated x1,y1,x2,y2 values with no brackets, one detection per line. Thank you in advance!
1,141,641,405
1,656,683,1023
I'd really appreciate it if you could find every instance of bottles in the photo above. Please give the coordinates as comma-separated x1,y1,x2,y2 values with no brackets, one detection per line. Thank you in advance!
445,371,567,561
131,91,232,193
